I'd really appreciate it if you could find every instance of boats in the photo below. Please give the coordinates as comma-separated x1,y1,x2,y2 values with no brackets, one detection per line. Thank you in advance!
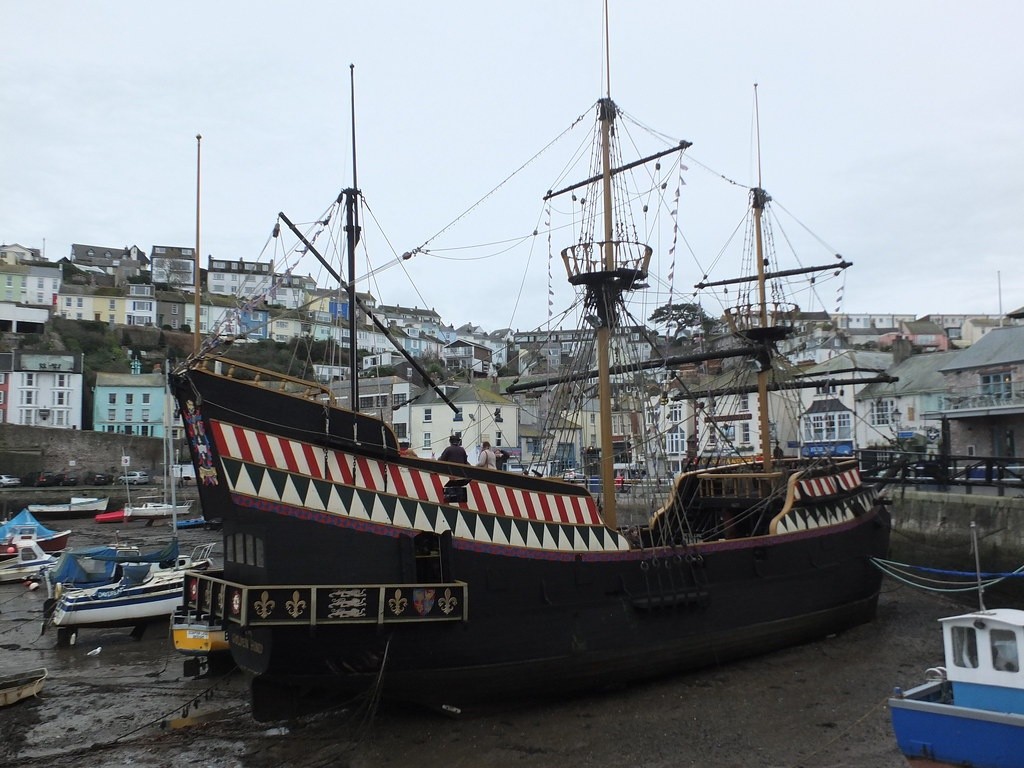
125,496,195,518
44,530,231,681
168,515,220,529
27,495,110,521
885,518,1024,768
0,668,48,707
0,508,72,560
168,0,891,723
0,541,61,586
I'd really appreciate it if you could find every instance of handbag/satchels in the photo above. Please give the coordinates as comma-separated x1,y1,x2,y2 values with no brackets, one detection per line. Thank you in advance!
478,450,488,468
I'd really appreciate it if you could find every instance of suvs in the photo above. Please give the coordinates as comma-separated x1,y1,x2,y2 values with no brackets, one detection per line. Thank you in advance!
119,472,150,485
23,471,55,488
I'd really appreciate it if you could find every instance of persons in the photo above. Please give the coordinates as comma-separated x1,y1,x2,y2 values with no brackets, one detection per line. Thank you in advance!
437,435,468,465
476,441,497,470
493,448,510,471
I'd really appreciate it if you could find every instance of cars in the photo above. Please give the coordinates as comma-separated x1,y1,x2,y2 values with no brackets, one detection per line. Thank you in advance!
85,473,109,487
54,474,78,486
0,475,22,488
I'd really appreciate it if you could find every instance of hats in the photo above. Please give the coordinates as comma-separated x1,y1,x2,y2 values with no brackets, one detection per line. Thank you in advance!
449,436,460,444
